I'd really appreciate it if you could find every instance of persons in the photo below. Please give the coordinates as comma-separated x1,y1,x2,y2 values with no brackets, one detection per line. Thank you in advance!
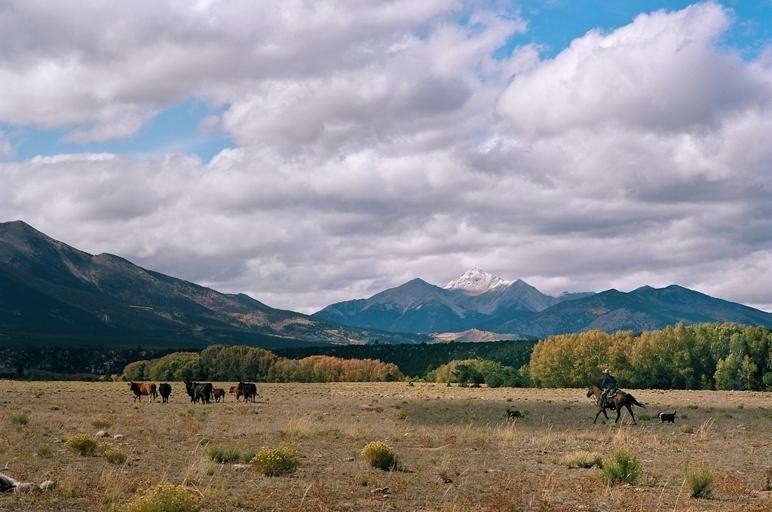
598,368,617,407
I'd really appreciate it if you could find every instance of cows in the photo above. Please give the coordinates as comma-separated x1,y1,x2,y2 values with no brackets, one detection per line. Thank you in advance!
183,378,261,403
159,383,172,403
128,380,158,403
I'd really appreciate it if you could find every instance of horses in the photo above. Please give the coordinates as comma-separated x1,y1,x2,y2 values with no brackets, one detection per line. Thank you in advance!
586,383,647,425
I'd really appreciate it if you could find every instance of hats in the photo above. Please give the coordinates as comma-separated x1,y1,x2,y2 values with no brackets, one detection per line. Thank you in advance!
601,368,612,374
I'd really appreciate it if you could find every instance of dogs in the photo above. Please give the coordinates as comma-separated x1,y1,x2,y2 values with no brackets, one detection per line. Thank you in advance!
657,410,677,425
505,409,525,423
